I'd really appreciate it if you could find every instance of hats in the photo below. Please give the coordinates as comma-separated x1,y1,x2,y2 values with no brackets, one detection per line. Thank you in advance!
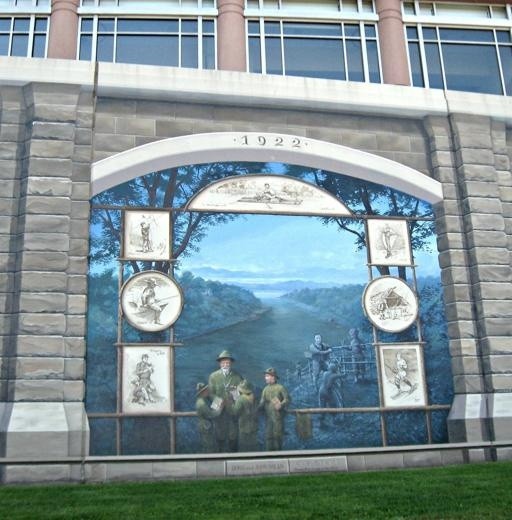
215,350,235,362
194,383,208,397
263,367,280,379
236,379,253,394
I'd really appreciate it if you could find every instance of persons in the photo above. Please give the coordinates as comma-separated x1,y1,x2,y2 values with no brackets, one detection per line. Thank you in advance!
340,327,367,383
131,352,157,405
308,331,329,389
141,222,152,253
194,381,223,452
260,182,274,199
208,350,243,453
392,352,414,393
224,379,257,453
380,223,392,256
253,366,291,451
140,278,165,326
316,364,346,426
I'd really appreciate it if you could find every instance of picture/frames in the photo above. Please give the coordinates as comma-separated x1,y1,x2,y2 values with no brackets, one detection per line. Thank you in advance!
118,206,182,417
186,172,353,217
361,217,431,409
